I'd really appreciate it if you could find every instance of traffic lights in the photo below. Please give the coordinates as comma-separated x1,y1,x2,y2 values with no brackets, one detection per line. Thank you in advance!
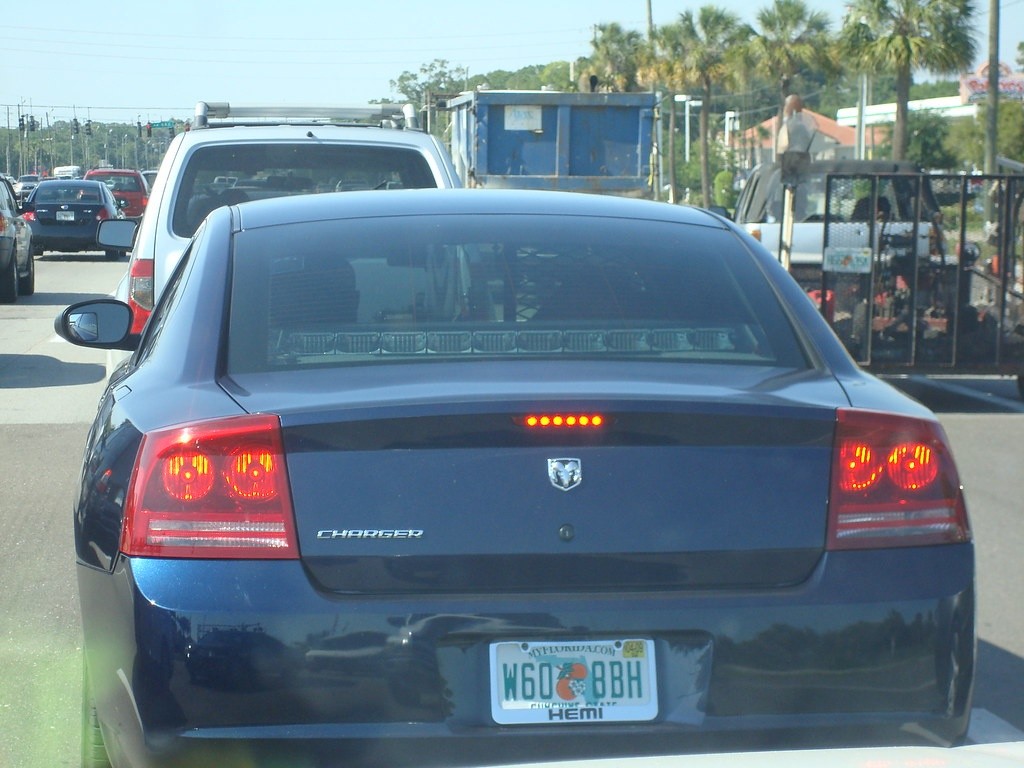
136,122,142,138
169,126,175,138
146,122,152,137
73,121,78,134
85,122,91,135
19,118,24,130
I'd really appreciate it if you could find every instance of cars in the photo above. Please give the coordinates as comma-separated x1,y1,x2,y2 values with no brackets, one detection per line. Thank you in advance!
49,183,976,767
23,178,130,261
0,179,36,304
0,166,160,305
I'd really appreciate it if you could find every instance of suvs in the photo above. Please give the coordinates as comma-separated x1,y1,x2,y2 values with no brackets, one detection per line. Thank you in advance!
107,101,485,366
706,157,980,308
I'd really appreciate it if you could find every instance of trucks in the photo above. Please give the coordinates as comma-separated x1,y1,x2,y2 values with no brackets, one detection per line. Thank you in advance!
433,80,665,202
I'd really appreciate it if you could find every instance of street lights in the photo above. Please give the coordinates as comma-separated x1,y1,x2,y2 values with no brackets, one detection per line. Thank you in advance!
668,83,692,205
682,100,704,204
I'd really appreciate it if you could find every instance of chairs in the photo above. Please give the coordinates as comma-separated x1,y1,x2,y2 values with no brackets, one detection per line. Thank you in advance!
187,189,249,227
81,194,98,202
851,196,894,221
114,183,140,192
528,268,686,320
